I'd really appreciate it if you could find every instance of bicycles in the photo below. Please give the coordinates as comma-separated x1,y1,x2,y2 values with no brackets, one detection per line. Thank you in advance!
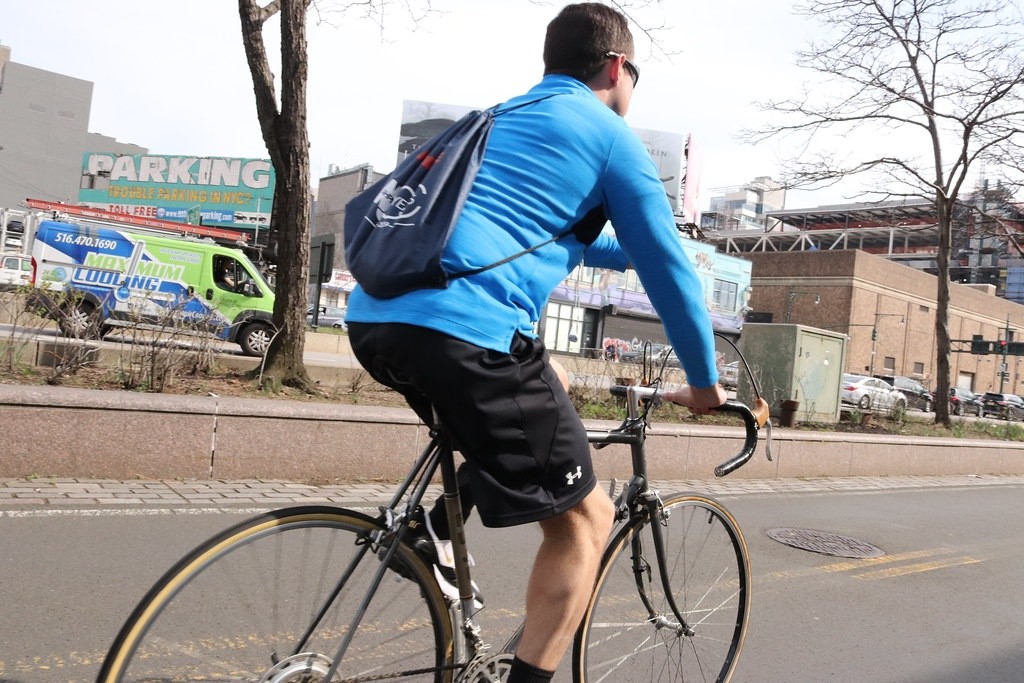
96,332,772,683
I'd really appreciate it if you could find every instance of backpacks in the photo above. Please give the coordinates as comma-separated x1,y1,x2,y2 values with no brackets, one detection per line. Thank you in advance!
339,88,608,302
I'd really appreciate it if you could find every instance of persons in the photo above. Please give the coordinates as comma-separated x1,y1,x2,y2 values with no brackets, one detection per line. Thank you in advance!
341,3,727,681
607,345,615,361
216,256,244,287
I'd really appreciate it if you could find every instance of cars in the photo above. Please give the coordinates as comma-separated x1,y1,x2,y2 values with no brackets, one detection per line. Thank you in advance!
620,344,682,367
841,373,907,413
718,360,739,390
305,304,346,329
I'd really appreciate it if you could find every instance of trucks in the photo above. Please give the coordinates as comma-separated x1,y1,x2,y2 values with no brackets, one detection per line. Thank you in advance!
0,212,276,357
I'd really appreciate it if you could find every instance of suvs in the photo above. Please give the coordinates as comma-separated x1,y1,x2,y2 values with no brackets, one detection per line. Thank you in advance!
873,374,1024,421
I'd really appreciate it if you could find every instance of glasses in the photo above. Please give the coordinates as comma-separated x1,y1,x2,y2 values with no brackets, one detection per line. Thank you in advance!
606,49,642,89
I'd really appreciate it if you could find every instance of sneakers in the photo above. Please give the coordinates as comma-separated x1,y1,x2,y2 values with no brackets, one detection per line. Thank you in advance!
376,524,487,612
